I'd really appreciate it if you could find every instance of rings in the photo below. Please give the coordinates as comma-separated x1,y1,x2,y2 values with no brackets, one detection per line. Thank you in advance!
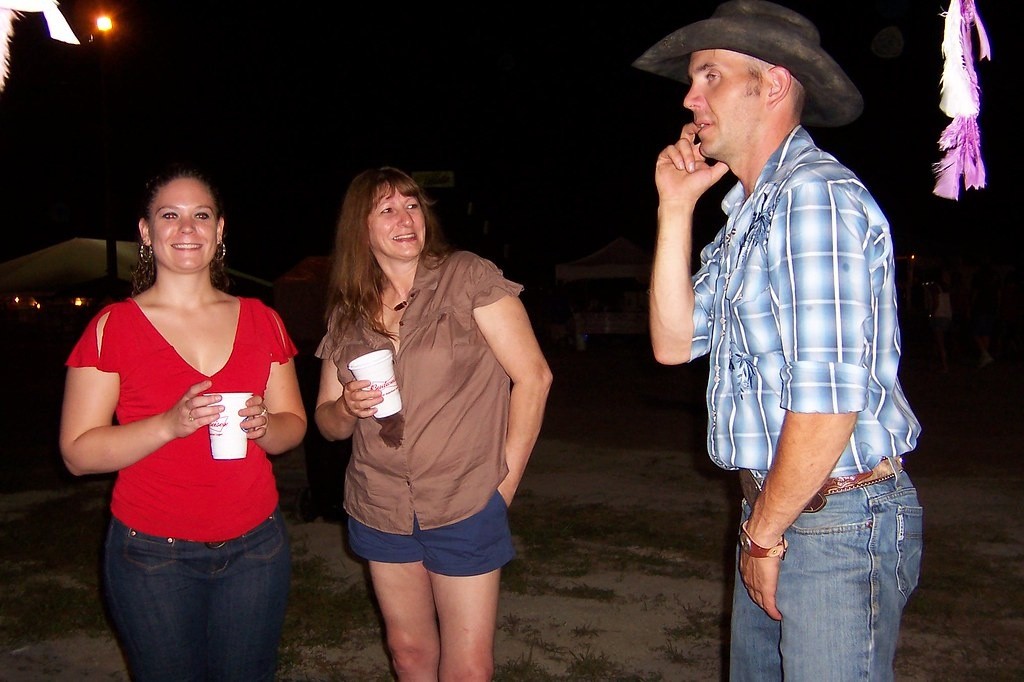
262,408,267,415
188,409,195,422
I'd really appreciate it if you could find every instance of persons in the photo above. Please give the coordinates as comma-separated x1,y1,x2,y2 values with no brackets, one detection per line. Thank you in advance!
313,165,555,682
632,1,925,682
59,163,306,682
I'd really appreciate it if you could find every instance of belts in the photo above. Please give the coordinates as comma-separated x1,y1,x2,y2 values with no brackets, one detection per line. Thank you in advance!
739,456,904,507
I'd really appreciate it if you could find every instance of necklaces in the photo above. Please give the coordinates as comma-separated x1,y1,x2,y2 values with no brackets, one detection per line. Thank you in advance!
381,288,412,312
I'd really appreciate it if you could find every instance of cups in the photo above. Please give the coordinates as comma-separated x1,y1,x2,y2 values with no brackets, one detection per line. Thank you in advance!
347,349,403,418
203,392,253,460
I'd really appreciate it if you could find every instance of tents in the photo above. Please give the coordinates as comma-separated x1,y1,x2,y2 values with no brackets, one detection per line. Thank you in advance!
553,236,651,310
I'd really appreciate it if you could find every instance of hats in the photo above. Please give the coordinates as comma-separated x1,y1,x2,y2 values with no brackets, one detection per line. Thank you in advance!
631,0,863,129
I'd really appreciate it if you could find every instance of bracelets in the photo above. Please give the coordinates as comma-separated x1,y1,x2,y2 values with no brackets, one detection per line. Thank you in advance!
739,519,788,561
